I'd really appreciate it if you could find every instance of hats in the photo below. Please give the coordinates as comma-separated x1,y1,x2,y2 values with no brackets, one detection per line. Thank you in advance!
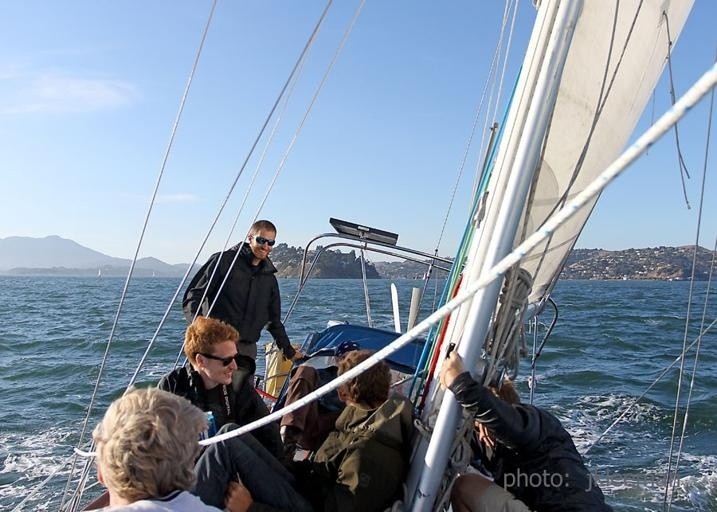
335,341,359,357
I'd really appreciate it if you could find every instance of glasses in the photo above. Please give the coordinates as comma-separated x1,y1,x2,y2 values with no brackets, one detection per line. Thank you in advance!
256,237,275,246
198,353,239,366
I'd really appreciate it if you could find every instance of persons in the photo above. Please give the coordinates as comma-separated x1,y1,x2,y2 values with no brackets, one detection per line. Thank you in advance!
84,386,223,512
439,350,615,512
277,340,361,464
187,350,414,512
151,316,281,453
182,218,306,388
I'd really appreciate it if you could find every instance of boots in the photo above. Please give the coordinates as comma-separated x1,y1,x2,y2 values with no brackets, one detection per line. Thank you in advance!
280,425,303,460
